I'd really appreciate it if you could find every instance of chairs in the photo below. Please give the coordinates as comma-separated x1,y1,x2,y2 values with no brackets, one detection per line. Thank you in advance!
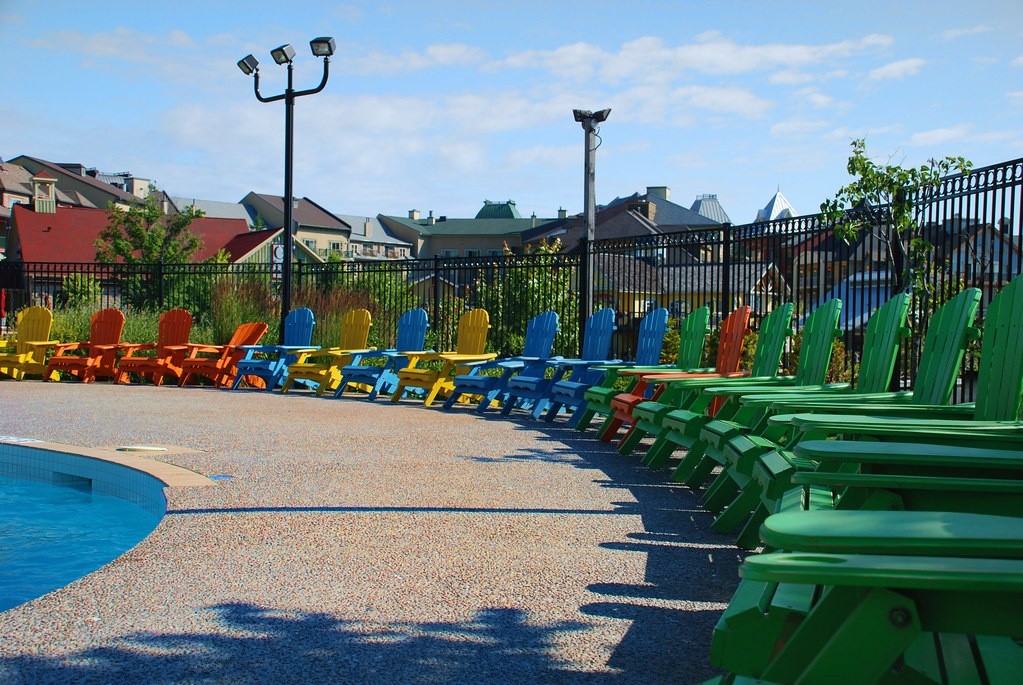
0,277,1023,685
178,321,266,389
